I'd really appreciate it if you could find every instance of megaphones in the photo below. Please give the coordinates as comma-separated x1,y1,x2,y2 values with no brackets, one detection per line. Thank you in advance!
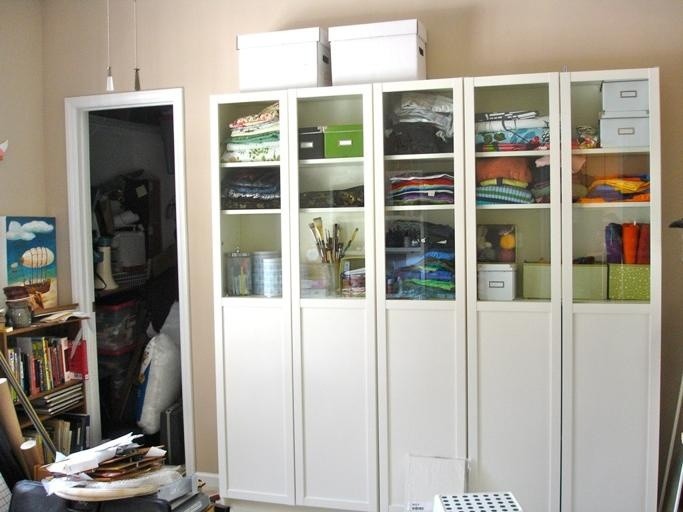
93,237,119,291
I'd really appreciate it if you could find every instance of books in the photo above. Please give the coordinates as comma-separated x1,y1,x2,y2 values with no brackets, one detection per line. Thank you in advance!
9,334,91,476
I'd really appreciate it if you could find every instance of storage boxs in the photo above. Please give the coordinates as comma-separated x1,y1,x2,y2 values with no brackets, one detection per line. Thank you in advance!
236,19,428,91
297,122,363,160
598,80,647,150
477,262,649,303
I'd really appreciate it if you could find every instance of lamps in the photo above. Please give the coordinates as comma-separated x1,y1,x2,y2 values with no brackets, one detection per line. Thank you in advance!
105,2,115,93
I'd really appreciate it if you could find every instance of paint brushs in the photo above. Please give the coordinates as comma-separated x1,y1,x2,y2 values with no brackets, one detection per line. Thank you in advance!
308,217,359,264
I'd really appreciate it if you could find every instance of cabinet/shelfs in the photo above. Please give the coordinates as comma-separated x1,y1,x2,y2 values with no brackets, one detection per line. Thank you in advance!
1,315,86,479
213,68,661,512
